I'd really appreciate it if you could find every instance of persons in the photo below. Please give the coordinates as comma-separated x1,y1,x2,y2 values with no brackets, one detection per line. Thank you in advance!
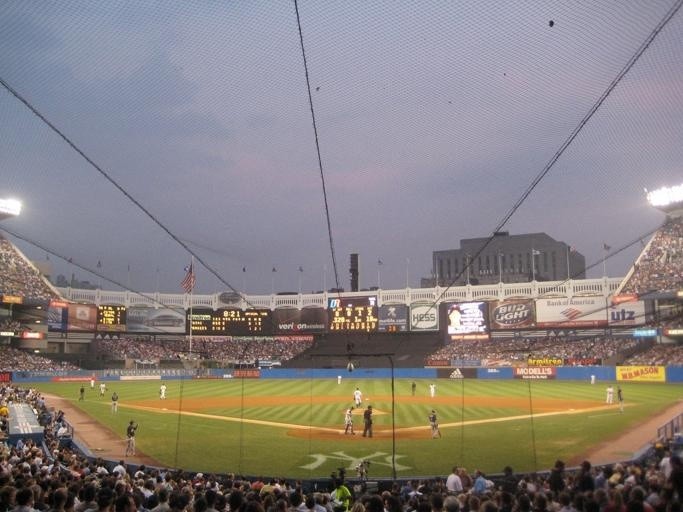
343,407,355,435
81,429,683,512
611,210,682,365
0,232,83,377
428,383,436,397
89,306,315,376
411,381,416,394
361,405,372,437
427,321,610,368
352,388,361,407
616,385,624,413
78,377,108,403
158,383,166,399
122,419,138,456
0,381,80,511
111,391,117,413
427,410,439,439
605,384,613,403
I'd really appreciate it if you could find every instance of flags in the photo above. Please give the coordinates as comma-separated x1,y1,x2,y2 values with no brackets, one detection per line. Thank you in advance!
180,262,196,293
